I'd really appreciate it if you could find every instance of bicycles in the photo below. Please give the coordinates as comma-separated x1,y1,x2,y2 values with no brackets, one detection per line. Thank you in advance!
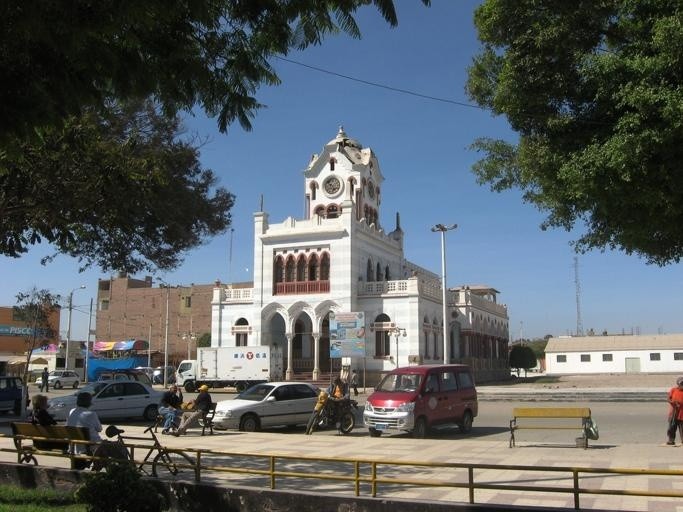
92,415,197,481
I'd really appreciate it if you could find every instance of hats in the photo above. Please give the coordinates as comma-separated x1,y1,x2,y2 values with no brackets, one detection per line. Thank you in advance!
198,385,208,391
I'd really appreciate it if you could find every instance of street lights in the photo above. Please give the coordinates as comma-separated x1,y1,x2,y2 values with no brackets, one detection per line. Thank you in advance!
429,223,457,380
156,275,171,390
181,328,197,360
64,285,85,371
387,326,407,368
518,319,522,347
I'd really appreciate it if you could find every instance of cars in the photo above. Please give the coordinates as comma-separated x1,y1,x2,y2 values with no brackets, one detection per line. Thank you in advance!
0,376,30,416
34,369,80,389
198,381,321,431
45,380,168,421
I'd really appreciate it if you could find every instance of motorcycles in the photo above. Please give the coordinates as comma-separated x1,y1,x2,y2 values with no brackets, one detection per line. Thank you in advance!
305,385,358,435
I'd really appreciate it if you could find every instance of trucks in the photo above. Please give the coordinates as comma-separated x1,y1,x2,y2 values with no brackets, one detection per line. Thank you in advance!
174,344,283,393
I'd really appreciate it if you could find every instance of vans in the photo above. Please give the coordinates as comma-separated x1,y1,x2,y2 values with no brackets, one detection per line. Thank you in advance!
361,363,477,439
98,364,177,392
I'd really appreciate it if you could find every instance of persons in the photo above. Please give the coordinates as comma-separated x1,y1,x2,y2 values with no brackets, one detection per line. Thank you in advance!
41,367,51,394
351,368,360,398
67,392,130,468
173,384,212,437
157,384,183,434
26,392,69,454
666,376,683,445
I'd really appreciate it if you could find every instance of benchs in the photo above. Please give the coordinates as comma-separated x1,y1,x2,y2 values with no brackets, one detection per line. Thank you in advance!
150,402,217,438
505,406,593,450
10,420,94,469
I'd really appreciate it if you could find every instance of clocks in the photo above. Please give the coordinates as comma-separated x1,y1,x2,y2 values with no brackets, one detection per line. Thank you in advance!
320,174,343,199
